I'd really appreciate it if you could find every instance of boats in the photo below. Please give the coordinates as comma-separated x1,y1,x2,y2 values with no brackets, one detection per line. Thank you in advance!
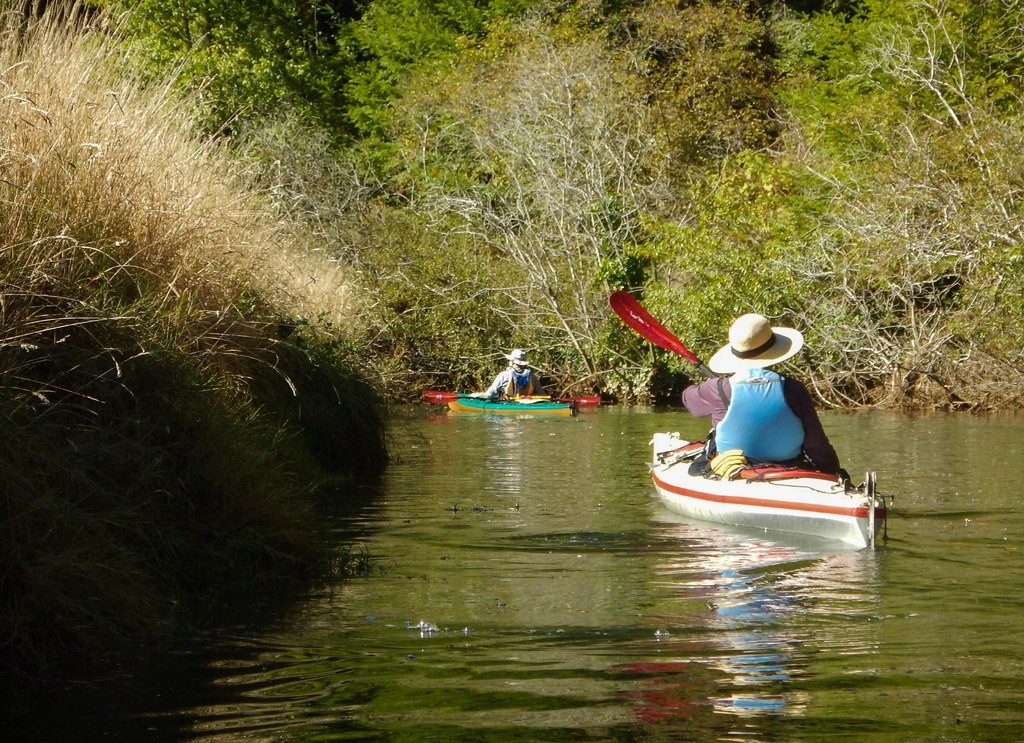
447,388,577,418
648,426,888,552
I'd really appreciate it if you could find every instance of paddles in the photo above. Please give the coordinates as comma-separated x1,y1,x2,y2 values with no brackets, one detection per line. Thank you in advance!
422,390,602,408
610,289,720,380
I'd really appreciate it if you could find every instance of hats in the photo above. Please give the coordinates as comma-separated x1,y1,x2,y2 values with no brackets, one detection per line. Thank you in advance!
708,314,805,373
504,348,533,366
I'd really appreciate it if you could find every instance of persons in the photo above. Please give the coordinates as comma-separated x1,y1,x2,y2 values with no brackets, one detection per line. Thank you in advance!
480,350,546,398
682,313,851,481
